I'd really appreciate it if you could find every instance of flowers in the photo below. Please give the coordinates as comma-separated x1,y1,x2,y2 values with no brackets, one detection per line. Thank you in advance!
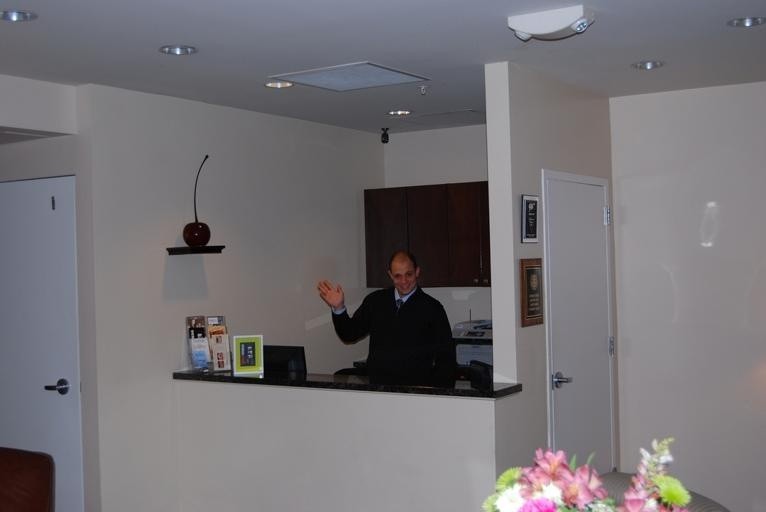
481,435,693,512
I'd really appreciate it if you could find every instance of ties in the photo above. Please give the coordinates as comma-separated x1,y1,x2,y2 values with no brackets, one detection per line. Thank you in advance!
396,299,404,309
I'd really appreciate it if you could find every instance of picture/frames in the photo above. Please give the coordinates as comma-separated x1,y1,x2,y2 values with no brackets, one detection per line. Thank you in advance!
521,259,544,326
519,194,539,243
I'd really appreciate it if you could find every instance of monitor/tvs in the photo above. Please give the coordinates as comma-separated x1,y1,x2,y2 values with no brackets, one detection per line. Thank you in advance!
264,345,308,382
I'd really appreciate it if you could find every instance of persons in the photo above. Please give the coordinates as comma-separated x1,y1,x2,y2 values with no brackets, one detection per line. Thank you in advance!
316,250,457,378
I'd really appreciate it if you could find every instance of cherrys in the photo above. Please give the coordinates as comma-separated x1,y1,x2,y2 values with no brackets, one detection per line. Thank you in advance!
183,155,211,247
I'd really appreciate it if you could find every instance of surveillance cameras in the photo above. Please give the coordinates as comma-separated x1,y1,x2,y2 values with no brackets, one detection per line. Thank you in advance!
381,127,389,143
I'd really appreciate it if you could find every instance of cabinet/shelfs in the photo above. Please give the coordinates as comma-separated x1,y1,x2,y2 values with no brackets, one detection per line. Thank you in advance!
363,180,492,289
